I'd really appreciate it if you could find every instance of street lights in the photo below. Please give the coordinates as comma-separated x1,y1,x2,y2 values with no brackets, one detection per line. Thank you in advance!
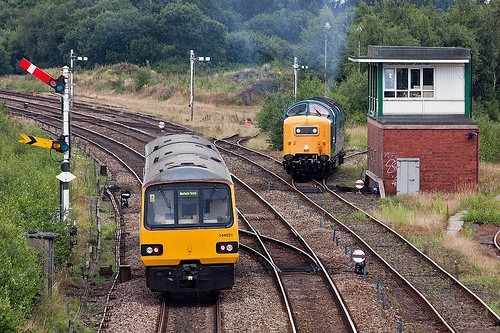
322,22,332,97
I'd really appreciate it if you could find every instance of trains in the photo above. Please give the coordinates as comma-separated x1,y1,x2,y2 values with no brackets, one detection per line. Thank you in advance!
281,96,347,180
139,134,240,294
